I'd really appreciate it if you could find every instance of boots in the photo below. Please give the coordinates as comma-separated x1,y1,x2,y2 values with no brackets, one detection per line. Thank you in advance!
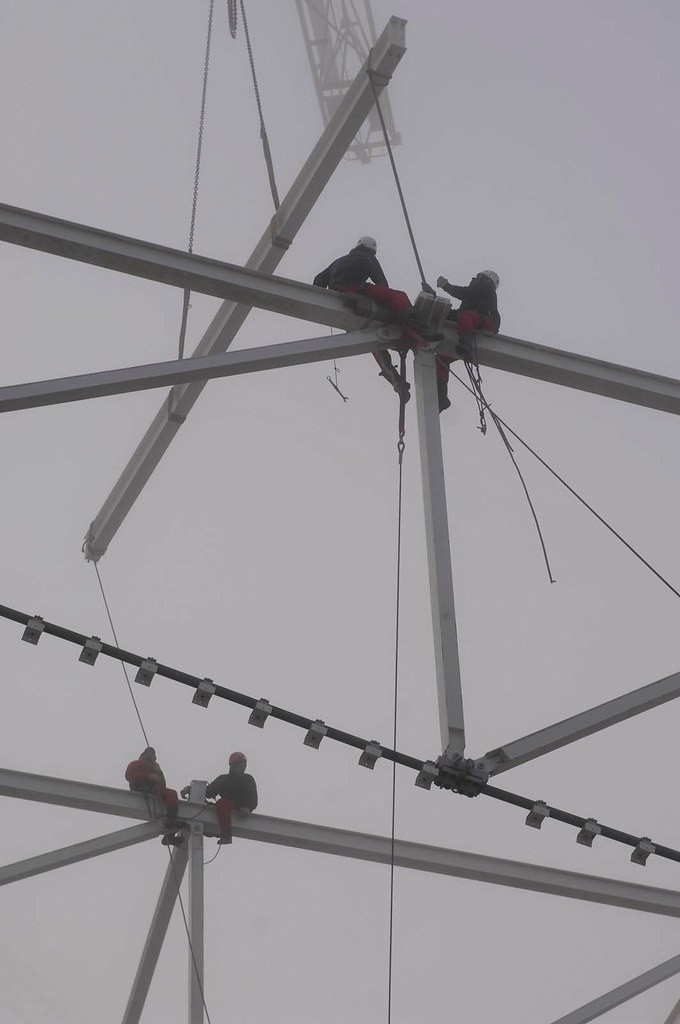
437,380,451,414
454,331,475,363
378,364,410,404
401,307,444,341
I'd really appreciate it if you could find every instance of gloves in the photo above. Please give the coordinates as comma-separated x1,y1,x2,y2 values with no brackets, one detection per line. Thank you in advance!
436,276,448,288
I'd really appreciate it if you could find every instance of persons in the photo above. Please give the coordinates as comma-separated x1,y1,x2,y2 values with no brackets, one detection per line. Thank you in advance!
314,236,445,404
437,270,500,414
125,747,185,846
181,752,258,844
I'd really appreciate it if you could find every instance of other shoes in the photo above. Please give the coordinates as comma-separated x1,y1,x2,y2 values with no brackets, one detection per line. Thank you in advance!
217,828,232,844
165,810,178,827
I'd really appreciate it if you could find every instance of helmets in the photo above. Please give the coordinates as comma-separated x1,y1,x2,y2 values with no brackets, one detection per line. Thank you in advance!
358,237,377,253
229,753,246,765
477,270,500,290
